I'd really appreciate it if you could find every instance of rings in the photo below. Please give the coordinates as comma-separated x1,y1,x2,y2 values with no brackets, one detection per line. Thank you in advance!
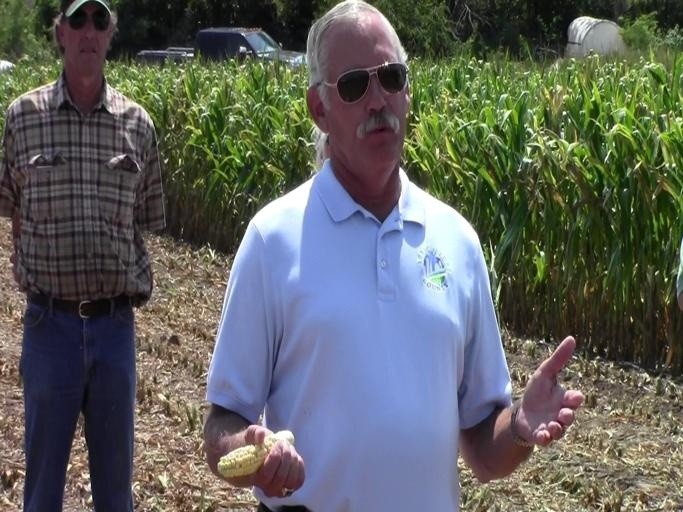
281,487,294,497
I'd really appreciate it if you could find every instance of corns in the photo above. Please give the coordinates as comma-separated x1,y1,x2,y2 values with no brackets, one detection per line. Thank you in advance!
215,426,295,478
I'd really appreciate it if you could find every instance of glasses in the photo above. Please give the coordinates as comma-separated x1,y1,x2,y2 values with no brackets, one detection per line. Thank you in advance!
60,0,112,18
69,10,110,31
317,62,409,104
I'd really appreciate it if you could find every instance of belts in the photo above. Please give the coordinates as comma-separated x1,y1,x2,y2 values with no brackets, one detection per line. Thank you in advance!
33,293,134,319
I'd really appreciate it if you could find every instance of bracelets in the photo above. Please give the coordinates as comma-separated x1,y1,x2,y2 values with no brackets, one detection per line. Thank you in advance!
511,398,534,449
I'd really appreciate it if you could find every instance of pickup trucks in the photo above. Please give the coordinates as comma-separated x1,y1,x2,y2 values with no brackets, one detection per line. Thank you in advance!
135,26,307,70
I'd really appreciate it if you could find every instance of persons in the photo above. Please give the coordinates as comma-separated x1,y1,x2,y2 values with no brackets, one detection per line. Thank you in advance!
0,0,167,512
203,0,586,512
